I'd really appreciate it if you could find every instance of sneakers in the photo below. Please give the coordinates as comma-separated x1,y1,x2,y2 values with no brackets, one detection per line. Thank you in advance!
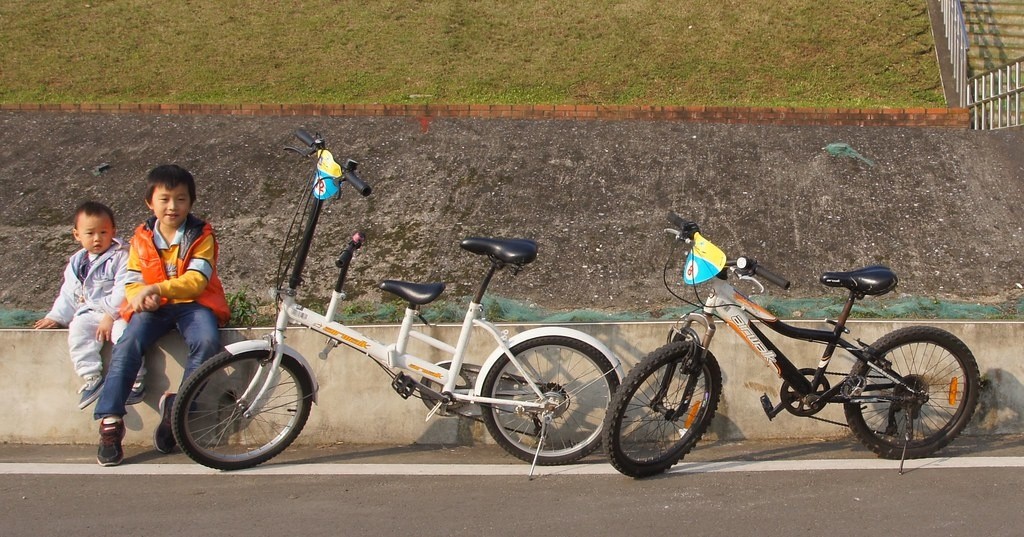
125,383,145,404
153,393,197,454
97,419,127,467
79,375,106,410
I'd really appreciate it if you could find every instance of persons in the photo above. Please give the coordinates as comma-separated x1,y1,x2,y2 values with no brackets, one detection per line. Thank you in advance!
34,201,147,410
94,164,230,467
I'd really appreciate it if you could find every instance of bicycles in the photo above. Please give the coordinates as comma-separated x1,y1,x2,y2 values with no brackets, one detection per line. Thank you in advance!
602,210,980,480
170,126,624,481
